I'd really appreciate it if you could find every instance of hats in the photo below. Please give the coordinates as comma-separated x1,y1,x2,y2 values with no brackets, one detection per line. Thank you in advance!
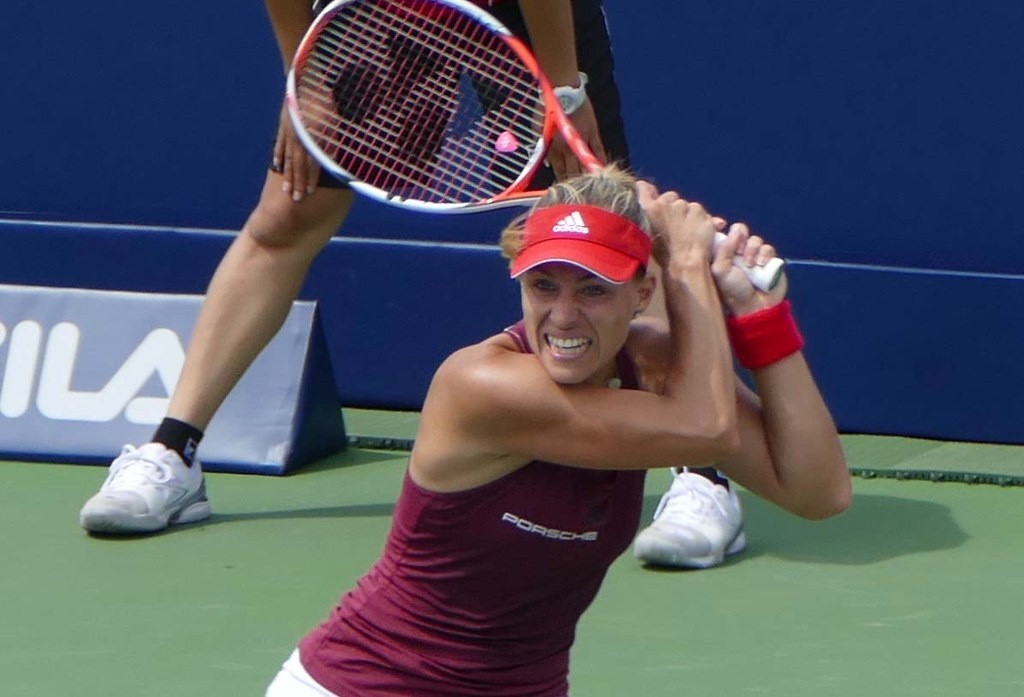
509,205,651,285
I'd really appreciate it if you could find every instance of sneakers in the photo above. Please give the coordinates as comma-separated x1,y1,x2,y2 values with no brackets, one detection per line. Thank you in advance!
634,466,749,569
79,442,210,534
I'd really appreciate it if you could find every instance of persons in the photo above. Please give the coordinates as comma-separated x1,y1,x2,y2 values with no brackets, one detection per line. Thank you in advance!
264,162,852,697
79,0,747,567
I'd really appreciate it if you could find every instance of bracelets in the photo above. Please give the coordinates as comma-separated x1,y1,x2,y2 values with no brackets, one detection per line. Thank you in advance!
728,298,804,374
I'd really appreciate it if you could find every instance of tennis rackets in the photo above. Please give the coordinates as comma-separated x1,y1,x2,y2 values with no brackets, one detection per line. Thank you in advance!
284,0,787,295
